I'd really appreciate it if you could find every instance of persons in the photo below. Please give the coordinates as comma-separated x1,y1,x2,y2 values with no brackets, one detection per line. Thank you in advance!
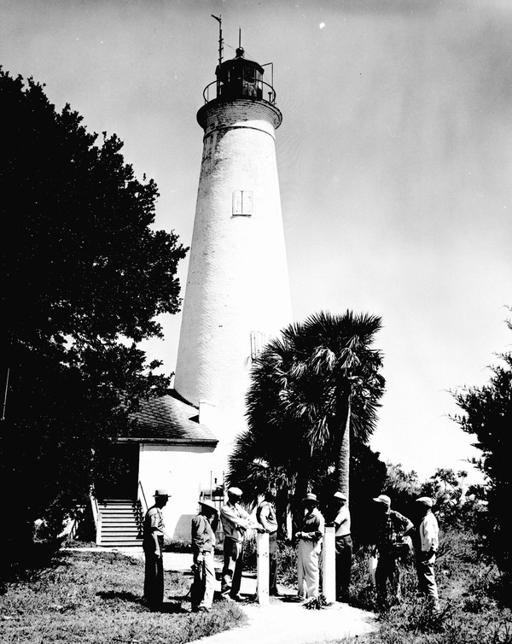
256,487,280,596
330,492,353,602
373,495,414,613
295,493,325,606
142,489,172,612
220,487,264,602
191,500,220,612
416,497,441,615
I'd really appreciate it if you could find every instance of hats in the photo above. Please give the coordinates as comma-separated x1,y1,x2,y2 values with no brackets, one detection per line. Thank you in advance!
229,486,243,495
416,496,432,508
372,494,391,506
333,492,348,502
197,499,218,511
153,489,172,498
302,492,321,504
